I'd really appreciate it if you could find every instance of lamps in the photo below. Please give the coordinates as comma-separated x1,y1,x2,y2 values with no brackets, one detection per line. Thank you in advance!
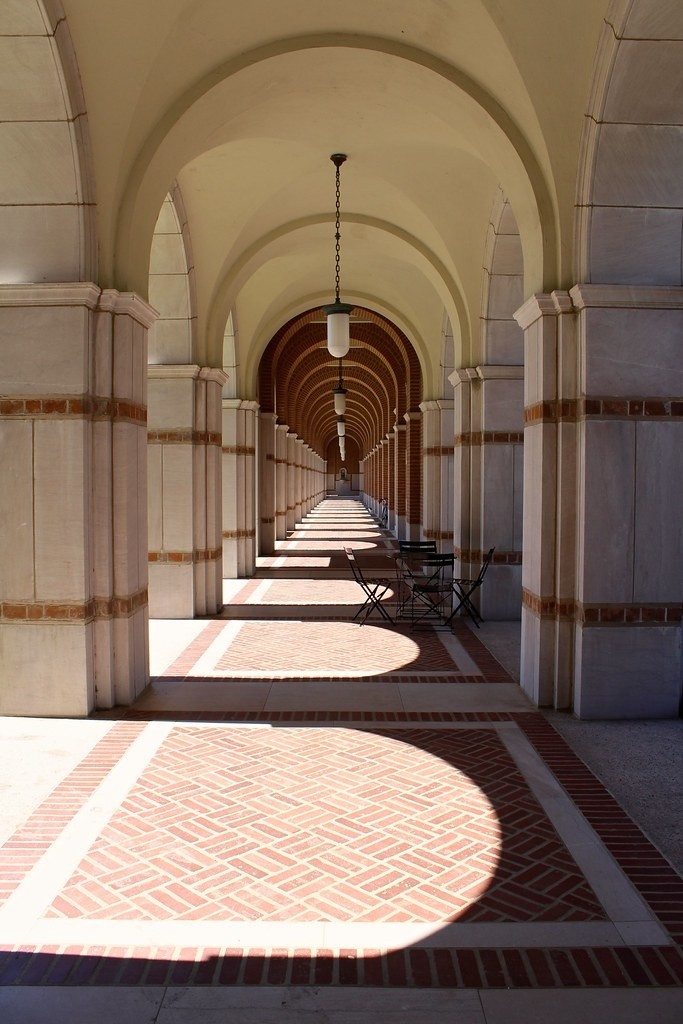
337,415,345,436
322,153,354,358
332,358,347,416
339,436,345,461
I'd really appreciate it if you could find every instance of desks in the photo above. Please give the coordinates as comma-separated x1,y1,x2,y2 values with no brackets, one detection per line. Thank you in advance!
387,552,457,626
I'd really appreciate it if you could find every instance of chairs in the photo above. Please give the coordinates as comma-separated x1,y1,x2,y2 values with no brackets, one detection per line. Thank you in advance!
398,539,446,618
342,547,397,627
442,545,497,629
410,552,452,632
